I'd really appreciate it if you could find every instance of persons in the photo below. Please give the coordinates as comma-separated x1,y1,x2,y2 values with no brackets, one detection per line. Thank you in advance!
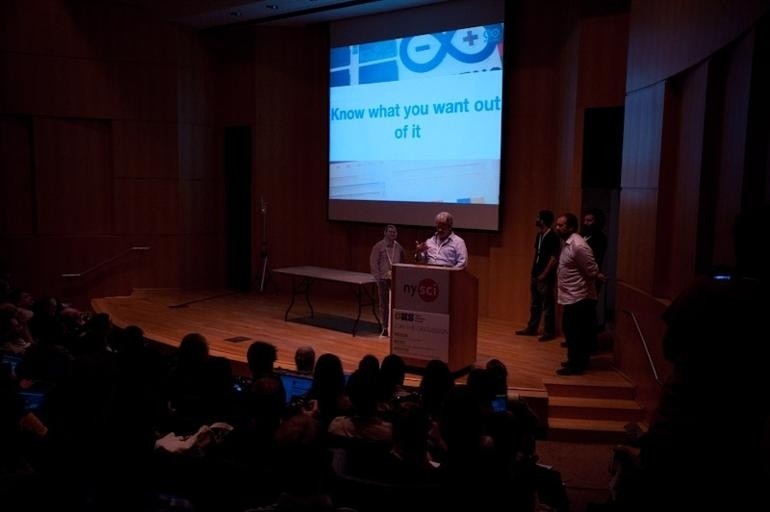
1,288,573,512
514,208,563,343
560,209,611,356
368,223,407,336
603,277,768,512
555,214,608,378
410,211,471,274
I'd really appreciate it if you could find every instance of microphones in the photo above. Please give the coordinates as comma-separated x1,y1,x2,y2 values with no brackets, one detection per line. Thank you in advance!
258,194,266,213
415,239,425,259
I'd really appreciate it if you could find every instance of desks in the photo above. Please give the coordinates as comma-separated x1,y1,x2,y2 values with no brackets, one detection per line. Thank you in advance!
271,265,382,337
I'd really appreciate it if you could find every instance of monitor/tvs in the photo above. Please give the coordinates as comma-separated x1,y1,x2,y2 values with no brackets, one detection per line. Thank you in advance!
280,372,315,404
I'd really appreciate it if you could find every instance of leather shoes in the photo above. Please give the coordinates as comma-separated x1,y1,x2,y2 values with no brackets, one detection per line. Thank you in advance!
516,328,537,336
539,334,555,342
382,329,388,336
561,342,568,347
556,367,584,375
561,361,592,370
